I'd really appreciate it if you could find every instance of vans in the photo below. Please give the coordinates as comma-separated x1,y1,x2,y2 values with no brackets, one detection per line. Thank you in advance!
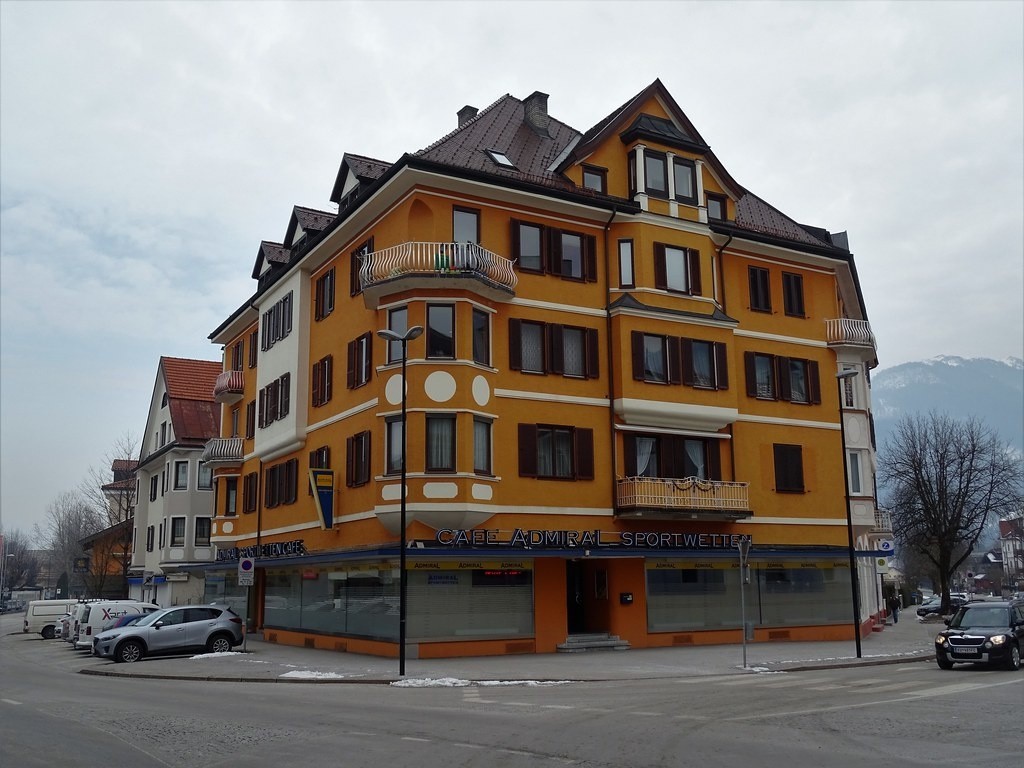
22,598,162,651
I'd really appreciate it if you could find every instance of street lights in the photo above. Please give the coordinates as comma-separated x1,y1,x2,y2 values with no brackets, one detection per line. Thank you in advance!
833,368,863,658
376,326,425,679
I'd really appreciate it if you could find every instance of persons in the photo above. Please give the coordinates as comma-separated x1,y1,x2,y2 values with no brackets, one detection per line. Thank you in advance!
888,594,902,624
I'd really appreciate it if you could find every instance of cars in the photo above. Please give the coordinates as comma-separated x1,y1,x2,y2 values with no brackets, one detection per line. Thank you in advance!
92,604,244,664
916,593,1024,670
